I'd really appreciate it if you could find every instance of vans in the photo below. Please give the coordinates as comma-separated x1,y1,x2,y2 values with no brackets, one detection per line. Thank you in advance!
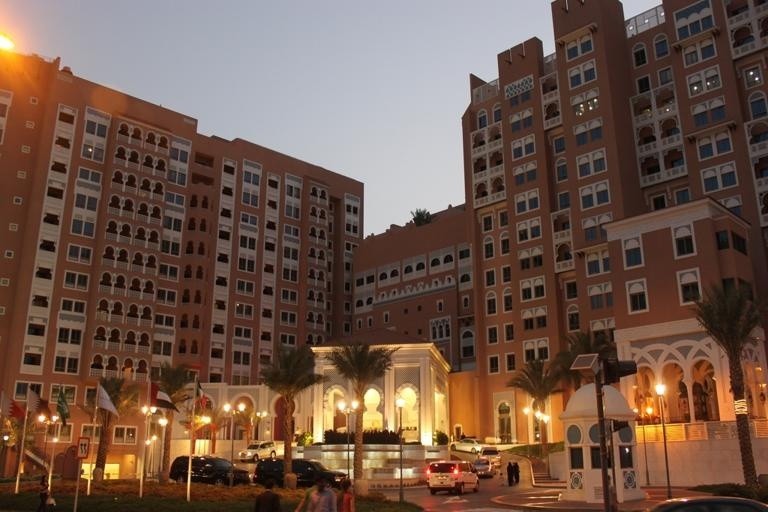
168,452,252,488
477,445,502,468
425,460,480,496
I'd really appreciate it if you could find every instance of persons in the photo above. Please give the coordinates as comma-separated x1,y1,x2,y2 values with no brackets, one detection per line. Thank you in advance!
256,481,280,512
507,462,513,486
295,478,355,512
512,462,519,483
38,475,49,512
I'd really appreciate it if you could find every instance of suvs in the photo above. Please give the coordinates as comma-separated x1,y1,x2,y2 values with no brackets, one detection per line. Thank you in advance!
251,458,347,489
238,440,276,463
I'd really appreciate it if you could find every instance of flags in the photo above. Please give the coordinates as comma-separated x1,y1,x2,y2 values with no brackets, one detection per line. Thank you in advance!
56,391,71,426
194,378,205,416
28,390,51,420
150,382,179,412
98,384,119,416
1,394,24,416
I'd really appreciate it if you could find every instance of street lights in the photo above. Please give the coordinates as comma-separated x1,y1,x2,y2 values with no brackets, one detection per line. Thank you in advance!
221,403,245,468
46,438,60,494
652,383,675,500
395,397,406,506
569,353,636,512
138,404,158,498
632,406,653,487
186,416,214,503
522,406,531,459
0,435,9,472
34,414,59,461
335,399,359,481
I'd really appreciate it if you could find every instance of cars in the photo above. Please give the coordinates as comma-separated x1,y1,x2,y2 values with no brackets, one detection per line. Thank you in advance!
641,493,767,512
448,437,490,455
470,457,496,479
